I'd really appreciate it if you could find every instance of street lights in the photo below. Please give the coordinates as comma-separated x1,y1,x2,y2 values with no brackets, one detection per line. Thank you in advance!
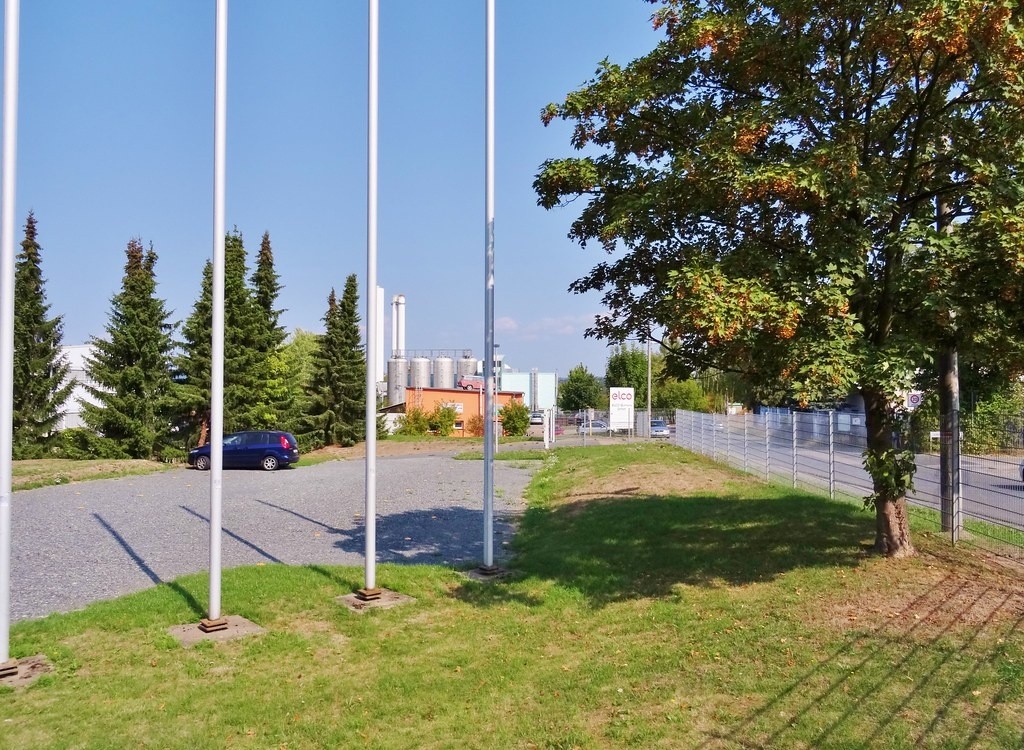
493,344,502,454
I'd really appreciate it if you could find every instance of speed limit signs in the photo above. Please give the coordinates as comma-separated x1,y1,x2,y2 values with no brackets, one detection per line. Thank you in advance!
910,394,920,404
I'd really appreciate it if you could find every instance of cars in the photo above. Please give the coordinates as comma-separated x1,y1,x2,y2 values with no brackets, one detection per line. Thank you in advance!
549,424,566,436
651,419,670,438
568,412,587,425
187,430,300,470
529,413,544,424
709,419,724,434
456,374,496,391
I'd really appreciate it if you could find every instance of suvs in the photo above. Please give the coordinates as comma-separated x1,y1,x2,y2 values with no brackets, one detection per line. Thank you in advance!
576,420,622,435
